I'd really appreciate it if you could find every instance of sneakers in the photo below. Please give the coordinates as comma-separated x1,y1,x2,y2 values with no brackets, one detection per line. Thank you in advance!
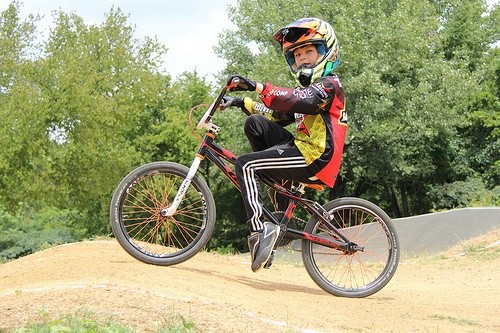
247,221,280,271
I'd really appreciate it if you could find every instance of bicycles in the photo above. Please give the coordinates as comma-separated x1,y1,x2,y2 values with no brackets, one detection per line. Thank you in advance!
108,77,401,298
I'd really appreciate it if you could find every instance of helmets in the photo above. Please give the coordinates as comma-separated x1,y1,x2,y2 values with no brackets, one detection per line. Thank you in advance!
274,18,341,89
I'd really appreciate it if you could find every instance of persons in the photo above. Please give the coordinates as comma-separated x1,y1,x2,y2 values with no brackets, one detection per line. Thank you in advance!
219,17,348,272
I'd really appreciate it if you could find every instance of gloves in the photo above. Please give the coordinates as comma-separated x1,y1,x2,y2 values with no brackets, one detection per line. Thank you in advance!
219,97,244,110
226,75,256,92
